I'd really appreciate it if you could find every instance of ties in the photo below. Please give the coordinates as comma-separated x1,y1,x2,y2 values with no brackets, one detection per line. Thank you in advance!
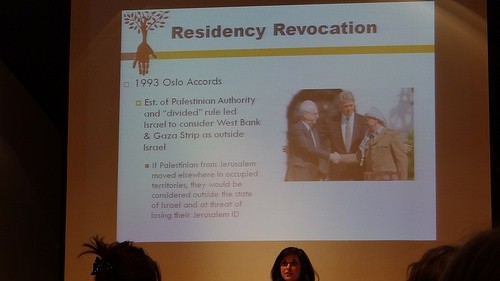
345,118,351,152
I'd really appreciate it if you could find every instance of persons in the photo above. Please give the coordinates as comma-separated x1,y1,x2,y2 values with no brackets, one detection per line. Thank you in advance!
76,232,163,281
283,99,343,181
404,245,460,281
269,246,321,281
280,90,370,181
332,106,410,181
436,227,500,281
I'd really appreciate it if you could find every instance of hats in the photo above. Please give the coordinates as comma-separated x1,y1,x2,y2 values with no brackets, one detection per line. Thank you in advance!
364,107,387,127
